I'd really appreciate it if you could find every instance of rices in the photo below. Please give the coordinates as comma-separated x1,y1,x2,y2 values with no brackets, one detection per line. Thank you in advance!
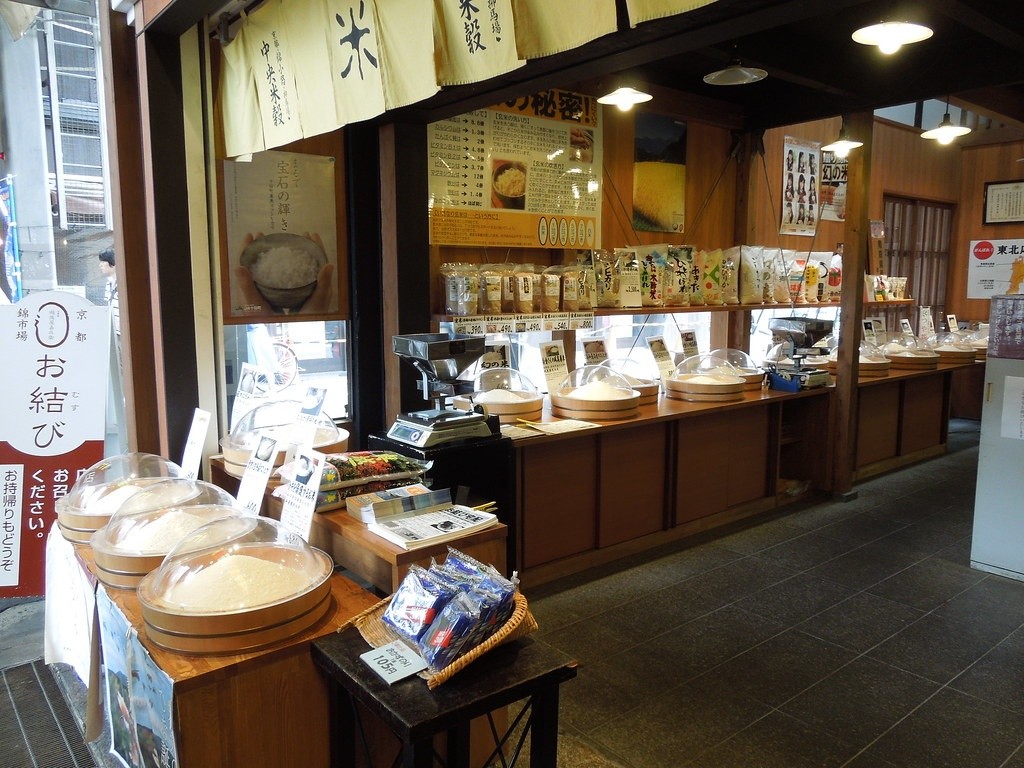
496,168,526,196
251,247,317,288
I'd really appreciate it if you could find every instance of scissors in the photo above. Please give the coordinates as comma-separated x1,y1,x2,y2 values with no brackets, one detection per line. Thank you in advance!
768,364,776,373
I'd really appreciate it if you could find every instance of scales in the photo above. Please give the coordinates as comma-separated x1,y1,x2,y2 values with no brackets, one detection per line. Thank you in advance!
386,333,493,448
768,317,834,393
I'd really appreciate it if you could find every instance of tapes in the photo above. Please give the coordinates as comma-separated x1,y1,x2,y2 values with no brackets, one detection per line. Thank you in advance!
778,372,791,382
474,404,488,421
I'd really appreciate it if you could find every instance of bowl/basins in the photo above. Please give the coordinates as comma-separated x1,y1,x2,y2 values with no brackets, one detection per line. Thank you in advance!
491,163,527,200
239,232,327,308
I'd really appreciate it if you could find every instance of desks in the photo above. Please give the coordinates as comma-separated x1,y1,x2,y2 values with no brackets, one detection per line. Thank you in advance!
309,628,580,768
56,518,384,767
208,453,507,596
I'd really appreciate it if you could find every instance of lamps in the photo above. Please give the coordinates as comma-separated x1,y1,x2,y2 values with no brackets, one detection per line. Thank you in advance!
852,21,933,55
920,94,972,144
821,116,863,158
703,38,768,86
596,68,653,111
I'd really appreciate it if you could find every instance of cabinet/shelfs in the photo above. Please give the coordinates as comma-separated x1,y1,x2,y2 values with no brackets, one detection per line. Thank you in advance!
38,9,114,243
429,299,986,603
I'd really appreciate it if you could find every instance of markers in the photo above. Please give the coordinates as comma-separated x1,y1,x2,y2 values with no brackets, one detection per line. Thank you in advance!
469,396,475,411
